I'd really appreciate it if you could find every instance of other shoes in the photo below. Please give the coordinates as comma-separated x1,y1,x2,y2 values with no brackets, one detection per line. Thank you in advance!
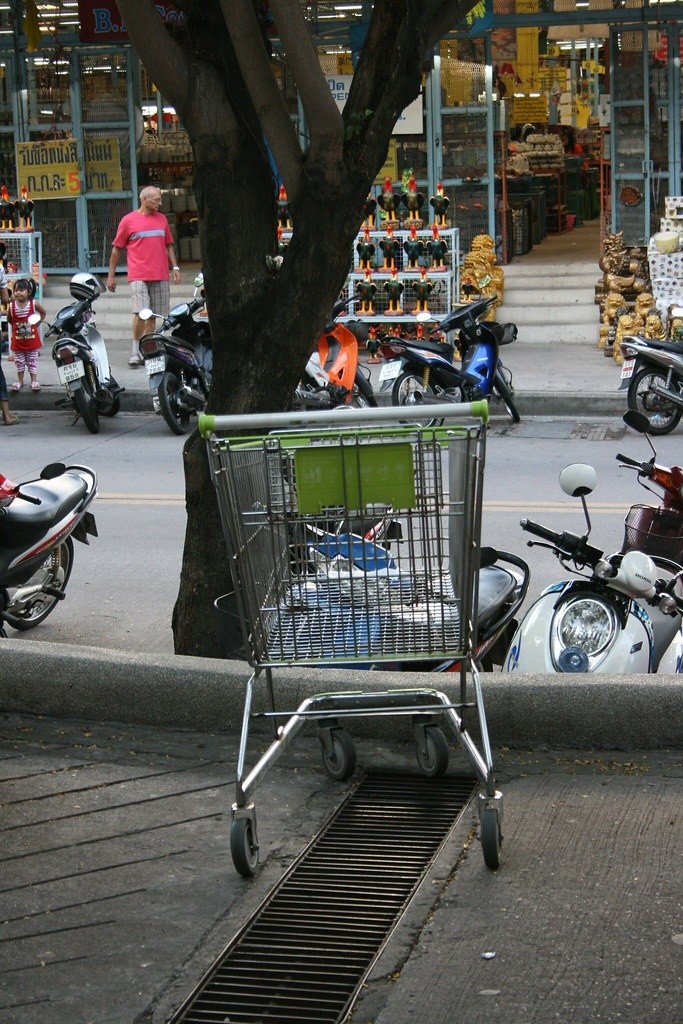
129,351,145,367
7,415,19,425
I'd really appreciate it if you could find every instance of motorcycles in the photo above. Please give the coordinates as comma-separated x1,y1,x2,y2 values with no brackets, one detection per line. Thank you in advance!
278,292,378,484
249,507,539,679
138,268,219,434
617,305,683,437
0,460,100,639
375,274,523,430
263,481,409,582
615,409,683,567
28,272,127,434
500,461,683,681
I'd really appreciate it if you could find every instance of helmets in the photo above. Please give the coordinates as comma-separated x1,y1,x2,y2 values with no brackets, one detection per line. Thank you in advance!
70,272,107,302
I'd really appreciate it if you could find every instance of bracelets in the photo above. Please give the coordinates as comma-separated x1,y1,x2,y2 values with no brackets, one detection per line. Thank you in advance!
172,266,180,270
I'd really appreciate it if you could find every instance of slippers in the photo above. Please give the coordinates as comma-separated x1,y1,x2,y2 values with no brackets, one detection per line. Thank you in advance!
10,382,21,390
30,382,40,390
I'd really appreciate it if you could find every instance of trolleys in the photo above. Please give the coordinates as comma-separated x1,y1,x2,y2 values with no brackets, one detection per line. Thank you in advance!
195,397,509,878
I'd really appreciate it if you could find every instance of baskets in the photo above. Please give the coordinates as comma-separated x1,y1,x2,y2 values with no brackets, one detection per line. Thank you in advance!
481,320,517,344
624,502,682,565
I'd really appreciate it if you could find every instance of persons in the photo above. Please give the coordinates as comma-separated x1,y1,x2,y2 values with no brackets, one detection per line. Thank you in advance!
107,186,183,366
0,240,20,424
562,132,589,169
6,277,46,391
518,123,537,147
